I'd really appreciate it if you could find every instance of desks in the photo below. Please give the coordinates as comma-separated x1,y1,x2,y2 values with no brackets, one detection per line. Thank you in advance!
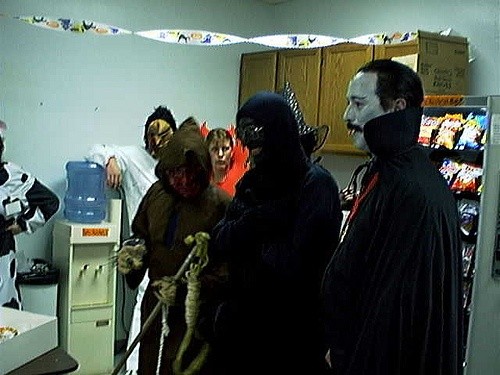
5,347,78,375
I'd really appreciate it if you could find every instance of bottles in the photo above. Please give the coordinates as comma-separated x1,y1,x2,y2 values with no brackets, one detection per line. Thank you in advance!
63,162,107,223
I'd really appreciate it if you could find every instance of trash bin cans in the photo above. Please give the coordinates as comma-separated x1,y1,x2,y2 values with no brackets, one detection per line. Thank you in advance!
15,258,60,317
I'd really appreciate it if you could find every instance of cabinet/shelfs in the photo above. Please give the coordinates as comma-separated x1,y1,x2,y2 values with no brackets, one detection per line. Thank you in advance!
238,30,469,158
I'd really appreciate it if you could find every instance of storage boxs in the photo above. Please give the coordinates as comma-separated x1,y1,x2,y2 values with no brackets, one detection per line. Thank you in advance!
0,306,58,375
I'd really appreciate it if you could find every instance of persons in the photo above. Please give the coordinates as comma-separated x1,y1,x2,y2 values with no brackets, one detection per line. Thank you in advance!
320,59,466,375
116,116,233,375
0,120,60,311
198,120,250,196
209,91,343,375
83,105,179,375
